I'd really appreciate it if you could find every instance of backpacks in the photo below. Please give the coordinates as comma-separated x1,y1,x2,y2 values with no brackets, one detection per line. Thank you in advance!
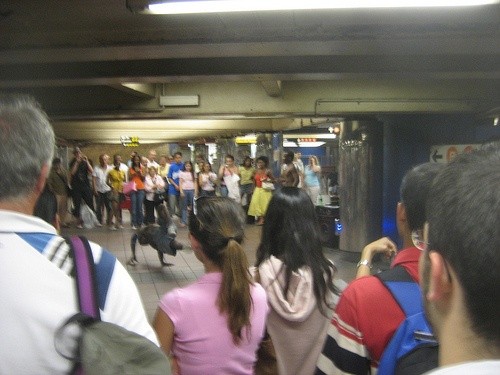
54,234,172,375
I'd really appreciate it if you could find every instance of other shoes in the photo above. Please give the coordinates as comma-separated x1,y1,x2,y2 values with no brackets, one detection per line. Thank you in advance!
116,224,125,230
77,224,82,229
131,224,136,231
256,221,264,226
109,224,117,231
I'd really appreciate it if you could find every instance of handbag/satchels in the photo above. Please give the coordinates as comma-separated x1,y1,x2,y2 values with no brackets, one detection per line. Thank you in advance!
122,181,137,194
261,169,275,191
65,186,72,197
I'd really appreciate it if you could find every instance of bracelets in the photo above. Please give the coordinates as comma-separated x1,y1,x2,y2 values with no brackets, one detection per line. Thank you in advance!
357,258,371,267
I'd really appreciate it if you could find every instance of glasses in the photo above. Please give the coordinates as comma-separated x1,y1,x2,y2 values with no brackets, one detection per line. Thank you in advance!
411,229,452,283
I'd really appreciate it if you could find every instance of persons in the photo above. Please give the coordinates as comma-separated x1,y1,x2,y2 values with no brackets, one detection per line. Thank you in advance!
48,146,322,231
34,191,60,232
0,103,160,375
249,186,349,375
152,196,268,375
128,205,191,264
356,237,396,279
417,141,500,375
314,162,447,375
